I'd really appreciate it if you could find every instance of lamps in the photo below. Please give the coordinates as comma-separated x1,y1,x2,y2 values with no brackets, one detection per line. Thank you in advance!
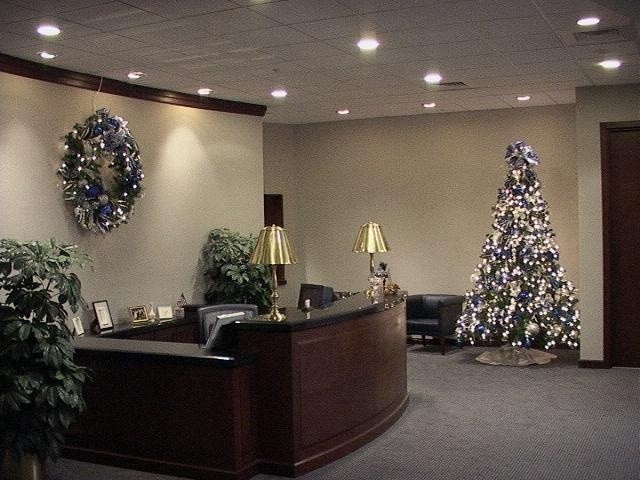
350,220,393,278
245,222,301,324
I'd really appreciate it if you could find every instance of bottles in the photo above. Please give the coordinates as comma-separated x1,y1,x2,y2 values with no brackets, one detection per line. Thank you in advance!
148,307,156,326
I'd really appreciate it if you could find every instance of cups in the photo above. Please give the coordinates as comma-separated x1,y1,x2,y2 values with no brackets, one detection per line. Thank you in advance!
305,299,310,308
175,308,185,319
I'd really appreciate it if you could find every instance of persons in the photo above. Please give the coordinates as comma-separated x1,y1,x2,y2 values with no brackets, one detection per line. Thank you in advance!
131,308,145,320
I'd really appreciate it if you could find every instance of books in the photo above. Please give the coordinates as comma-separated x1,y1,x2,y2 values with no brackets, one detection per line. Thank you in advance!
206,311,246,349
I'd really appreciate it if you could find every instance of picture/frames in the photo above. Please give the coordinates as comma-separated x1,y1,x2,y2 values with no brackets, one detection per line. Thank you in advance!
71,315,86,337
90,299,115,332
156,304,174,320
126,304,149,324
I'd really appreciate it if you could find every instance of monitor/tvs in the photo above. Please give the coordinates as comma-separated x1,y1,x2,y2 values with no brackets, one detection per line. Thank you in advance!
298,283,337,309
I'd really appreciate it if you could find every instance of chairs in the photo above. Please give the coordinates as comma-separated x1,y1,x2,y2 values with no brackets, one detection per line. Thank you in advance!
195,303,259,344
405,293,465,359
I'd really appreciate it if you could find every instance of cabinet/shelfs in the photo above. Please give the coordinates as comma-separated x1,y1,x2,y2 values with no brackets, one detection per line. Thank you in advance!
121,315,197,345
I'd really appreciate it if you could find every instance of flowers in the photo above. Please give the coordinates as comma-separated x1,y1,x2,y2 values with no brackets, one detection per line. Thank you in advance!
56,107,147,235
